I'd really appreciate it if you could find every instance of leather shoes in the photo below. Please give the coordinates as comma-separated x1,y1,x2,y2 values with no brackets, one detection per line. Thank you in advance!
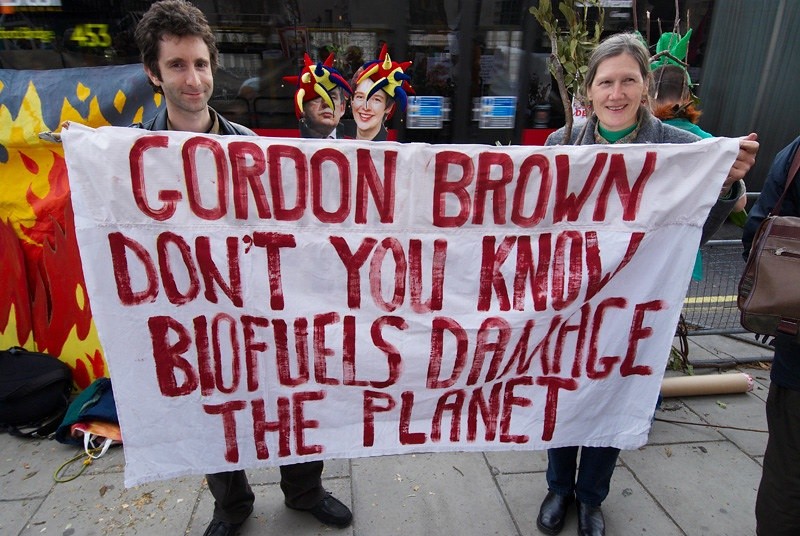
575,496,606,536
537,492,574,535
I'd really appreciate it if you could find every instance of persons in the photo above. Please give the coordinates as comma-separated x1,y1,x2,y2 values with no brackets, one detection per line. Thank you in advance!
741,133,800,536
281,48,354,139
644,30,748,279
124,0,354,536
537,32,758,536
348,42,414,141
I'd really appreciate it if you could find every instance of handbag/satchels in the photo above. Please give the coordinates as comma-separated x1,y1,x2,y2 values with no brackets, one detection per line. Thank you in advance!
51,374,123,482
736,145,800,341
0,350,74,440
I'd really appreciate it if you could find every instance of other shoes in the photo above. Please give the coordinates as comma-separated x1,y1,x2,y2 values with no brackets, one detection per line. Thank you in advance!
203,504,254,536
284,492,353,528
